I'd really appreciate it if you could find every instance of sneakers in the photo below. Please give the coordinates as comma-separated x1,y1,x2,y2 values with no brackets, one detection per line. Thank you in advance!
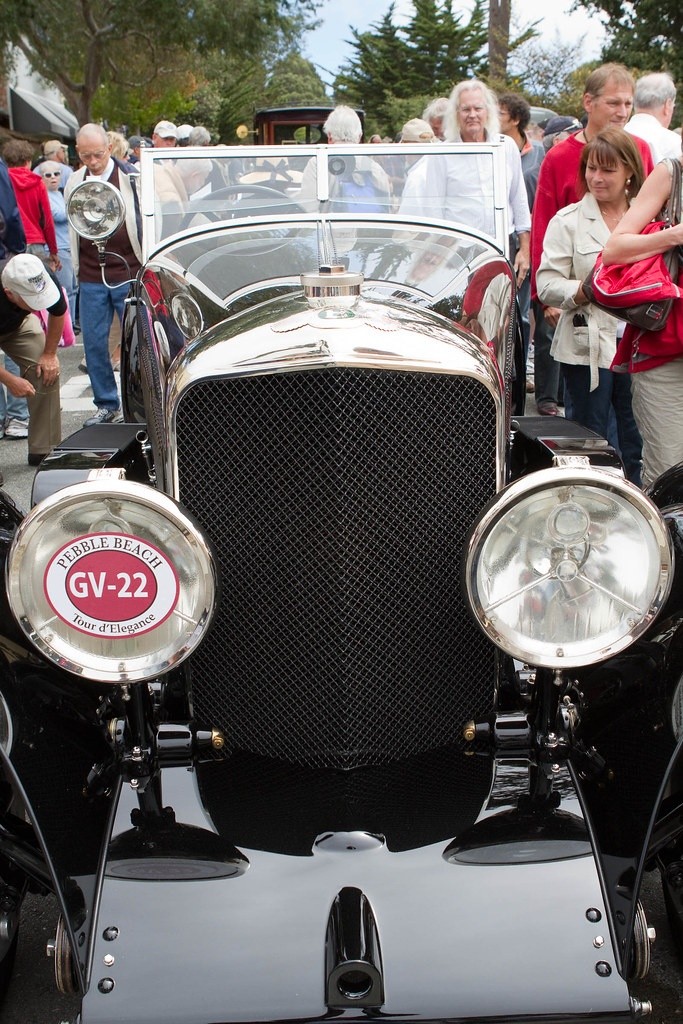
83,406,124,428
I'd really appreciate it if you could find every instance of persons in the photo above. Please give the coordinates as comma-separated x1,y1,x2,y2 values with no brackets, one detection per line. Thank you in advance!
321,228,358,269
458,261,513,328
0,121,246,486
405,234,472,284
465,271,513,357
292,62,683,490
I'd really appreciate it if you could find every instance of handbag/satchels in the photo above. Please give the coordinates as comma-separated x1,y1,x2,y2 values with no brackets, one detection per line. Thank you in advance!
30,286,75,348
580,158,681,332
332,166,388,213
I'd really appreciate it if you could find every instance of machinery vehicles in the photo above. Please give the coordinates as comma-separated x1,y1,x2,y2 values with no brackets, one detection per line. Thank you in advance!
236,106,367,145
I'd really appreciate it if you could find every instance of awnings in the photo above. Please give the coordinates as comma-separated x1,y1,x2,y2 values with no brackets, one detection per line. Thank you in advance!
7,86,80,139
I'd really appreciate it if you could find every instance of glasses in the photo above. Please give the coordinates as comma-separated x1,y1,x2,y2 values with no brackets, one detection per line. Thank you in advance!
555,122,583,132
41,172,60,180
79,148,108,161
123,152,128,159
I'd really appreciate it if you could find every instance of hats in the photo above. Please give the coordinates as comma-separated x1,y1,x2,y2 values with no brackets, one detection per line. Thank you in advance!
144,137,153,145
127,135,150,148
2,253,61,312
154,121,178,139
399,118,434,142
542,115,583,134
44,140,69,155
175,124,193,141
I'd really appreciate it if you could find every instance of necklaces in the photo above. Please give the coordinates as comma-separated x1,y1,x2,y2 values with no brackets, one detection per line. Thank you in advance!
600,207,628,222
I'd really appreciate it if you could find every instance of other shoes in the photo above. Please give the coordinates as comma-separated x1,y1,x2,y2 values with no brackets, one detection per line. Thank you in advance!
5,419,29,437
525,378,534,392
537,402,558,416
79,358,88,374
27,453,44,466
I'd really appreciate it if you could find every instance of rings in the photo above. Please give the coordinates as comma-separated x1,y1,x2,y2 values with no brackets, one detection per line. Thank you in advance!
57,372,60,375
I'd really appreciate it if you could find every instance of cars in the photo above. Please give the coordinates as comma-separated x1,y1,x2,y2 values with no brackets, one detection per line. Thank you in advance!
1,139,683,1024
530,105,559,127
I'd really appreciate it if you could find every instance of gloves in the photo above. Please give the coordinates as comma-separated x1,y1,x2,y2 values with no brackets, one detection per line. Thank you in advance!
573,313,587,327
